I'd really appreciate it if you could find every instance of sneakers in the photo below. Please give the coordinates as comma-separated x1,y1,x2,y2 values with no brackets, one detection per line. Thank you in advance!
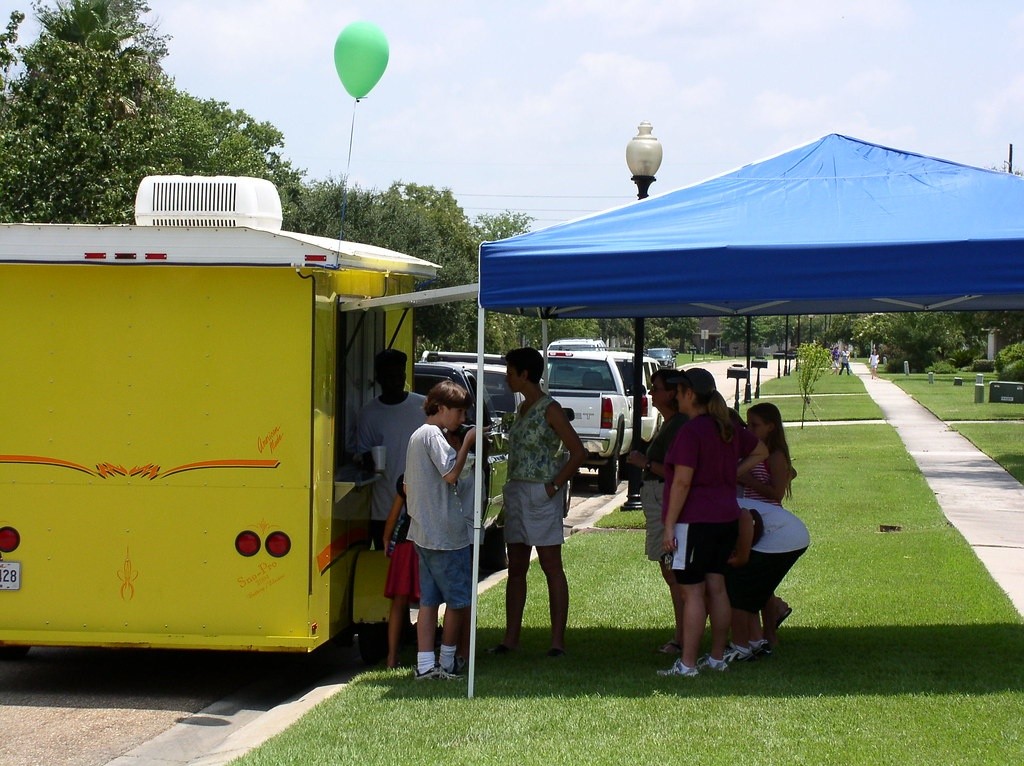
695,653,728,671
723,640,754,664
657,658,699,677
748,639,774,660
448,655,466,672
414,662,464,681
657,640,683,654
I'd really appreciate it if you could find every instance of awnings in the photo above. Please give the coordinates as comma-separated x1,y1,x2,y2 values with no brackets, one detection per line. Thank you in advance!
339,283,478,312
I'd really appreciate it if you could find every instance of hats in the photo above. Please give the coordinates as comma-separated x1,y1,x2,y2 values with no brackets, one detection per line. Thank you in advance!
833,346,839,349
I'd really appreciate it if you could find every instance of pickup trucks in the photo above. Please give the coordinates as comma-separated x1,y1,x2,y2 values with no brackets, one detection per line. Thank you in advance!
535,349,634,495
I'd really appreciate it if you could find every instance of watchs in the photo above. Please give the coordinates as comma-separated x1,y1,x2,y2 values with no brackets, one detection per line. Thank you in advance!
645,459,654,472
551,481,560,491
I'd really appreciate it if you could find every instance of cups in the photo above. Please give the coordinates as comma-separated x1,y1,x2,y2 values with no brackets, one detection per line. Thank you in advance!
371,446,386,473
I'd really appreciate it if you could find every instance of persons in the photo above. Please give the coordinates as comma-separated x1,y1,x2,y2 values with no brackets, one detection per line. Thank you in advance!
383,380,493,682
356,351,443,652
624,366,811,678
869,349,880,379
484,347,588,661
828,346,851,376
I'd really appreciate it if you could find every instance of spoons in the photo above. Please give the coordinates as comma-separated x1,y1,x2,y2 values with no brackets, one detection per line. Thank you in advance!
462,423,476,428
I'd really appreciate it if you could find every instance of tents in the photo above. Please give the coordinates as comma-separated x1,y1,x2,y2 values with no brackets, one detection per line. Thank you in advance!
468,132,1024,698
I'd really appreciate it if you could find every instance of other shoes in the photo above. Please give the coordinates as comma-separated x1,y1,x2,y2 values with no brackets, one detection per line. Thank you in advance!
545,647,568,665
484,644,514,656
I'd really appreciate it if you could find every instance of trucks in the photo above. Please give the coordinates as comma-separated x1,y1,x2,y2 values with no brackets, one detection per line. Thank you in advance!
0,174,440,652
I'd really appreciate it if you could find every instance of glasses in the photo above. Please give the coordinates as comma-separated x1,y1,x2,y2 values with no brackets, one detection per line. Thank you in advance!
678,369,695,389
835,348,839,349
650,385,663,392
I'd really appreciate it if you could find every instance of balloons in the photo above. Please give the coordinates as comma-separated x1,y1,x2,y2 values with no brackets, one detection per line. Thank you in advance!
333,19,389,101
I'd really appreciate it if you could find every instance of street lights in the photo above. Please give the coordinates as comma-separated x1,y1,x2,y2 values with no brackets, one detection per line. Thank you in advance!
621,121,663,511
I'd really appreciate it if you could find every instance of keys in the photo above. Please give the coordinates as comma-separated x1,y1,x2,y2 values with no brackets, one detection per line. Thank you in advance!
664,553,669,570
668,553,673,570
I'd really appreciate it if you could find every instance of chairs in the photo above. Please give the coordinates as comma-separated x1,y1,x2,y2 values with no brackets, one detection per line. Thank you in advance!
581,372,605,388
554,365,574,380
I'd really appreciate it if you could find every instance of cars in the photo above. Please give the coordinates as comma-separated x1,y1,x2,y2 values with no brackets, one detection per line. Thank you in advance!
410,362,508,574
416,344,573,518
646,346,676,371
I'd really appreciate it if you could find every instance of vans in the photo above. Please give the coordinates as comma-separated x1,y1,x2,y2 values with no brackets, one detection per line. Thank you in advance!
611,350,665,433
545,337,606,353
